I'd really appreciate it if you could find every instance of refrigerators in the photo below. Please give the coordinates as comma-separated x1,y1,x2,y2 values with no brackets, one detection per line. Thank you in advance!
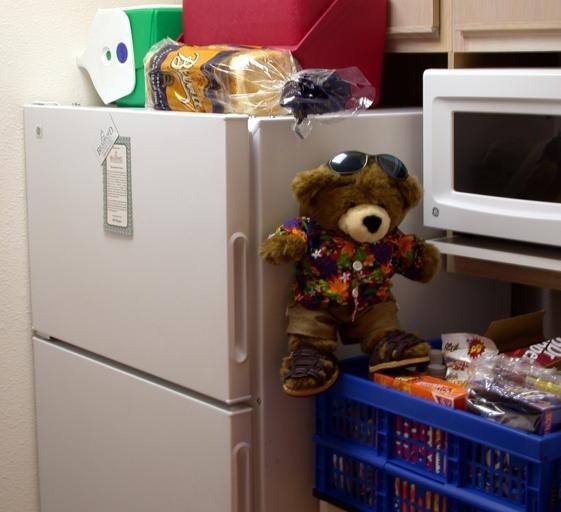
18,101,512,512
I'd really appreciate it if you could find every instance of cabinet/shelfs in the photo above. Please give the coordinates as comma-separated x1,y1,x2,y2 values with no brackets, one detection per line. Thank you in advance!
382,0,456,58
441,1,561,295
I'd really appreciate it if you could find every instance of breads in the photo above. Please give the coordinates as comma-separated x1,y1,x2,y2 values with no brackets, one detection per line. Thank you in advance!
144,36,298,117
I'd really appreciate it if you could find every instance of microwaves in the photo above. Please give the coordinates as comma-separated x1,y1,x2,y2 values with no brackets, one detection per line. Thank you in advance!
421,67,561,248
